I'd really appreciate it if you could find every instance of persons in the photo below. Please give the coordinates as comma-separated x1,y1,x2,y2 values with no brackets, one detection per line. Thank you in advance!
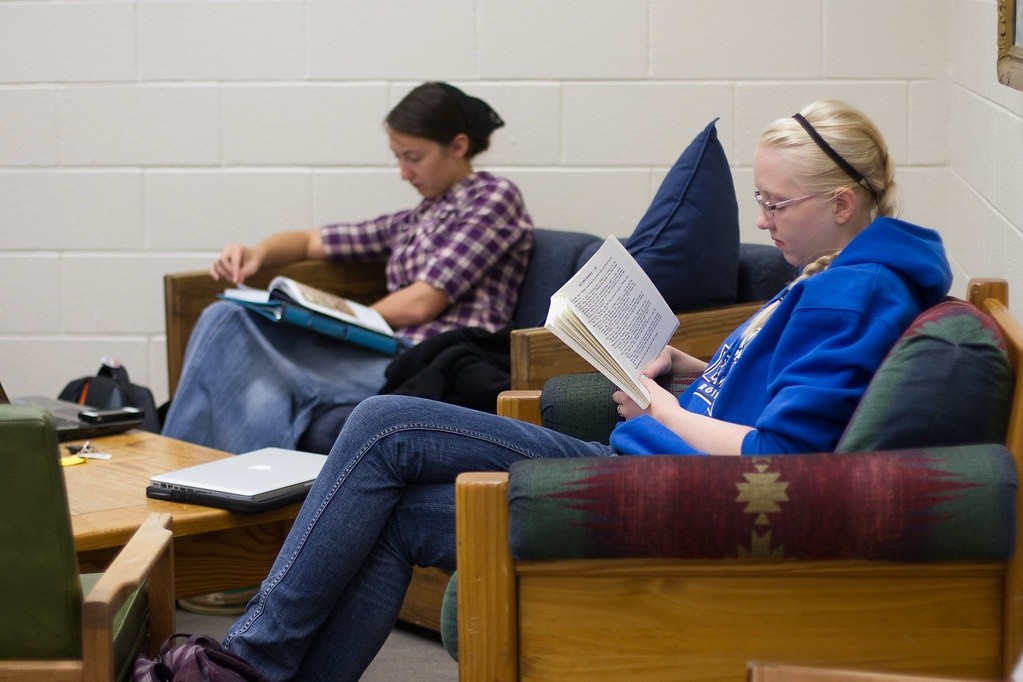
138,98,952,682
162,83,534,455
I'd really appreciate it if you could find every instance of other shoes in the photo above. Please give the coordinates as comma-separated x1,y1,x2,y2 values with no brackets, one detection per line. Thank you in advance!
178,586,260,616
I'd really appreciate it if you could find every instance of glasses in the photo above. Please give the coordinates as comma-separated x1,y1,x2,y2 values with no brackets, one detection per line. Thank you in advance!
753,181,833,218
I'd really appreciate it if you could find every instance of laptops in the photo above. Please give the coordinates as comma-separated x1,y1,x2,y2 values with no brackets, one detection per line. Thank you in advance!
149,448,328,505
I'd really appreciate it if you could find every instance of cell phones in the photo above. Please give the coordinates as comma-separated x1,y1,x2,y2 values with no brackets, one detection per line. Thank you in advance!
77,406,144,424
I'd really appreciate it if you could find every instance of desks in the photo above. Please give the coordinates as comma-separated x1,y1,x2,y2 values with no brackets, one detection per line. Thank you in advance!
59,429,303,600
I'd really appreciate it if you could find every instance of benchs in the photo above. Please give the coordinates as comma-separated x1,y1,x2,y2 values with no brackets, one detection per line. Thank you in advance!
164,230,805,633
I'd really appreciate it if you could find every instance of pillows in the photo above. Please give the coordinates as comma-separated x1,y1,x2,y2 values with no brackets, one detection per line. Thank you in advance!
626,117,741,315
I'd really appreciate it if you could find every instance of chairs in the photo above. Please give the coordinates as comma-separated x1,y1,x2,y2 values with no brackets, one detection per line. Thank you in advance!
439,277,1023,682
0,403,177,682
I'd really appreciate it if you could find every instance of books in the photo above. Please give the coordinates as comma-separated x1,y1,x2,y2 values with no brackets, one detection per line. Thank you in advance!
216,276,397,354
544,235,681,410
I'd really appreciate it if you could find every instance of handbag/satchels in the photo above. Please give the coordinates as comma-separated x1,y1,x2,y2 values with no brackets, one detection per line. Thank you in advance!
133,632,264,682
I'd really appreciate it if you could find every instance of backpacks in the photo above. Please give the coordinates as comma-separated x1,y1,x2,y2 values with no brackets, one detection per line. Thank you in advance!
58,361,161,432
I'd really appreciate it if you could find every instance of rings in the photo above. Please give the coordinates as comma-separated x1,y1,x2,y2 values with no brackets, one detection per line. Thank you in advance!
617,405,622,414
